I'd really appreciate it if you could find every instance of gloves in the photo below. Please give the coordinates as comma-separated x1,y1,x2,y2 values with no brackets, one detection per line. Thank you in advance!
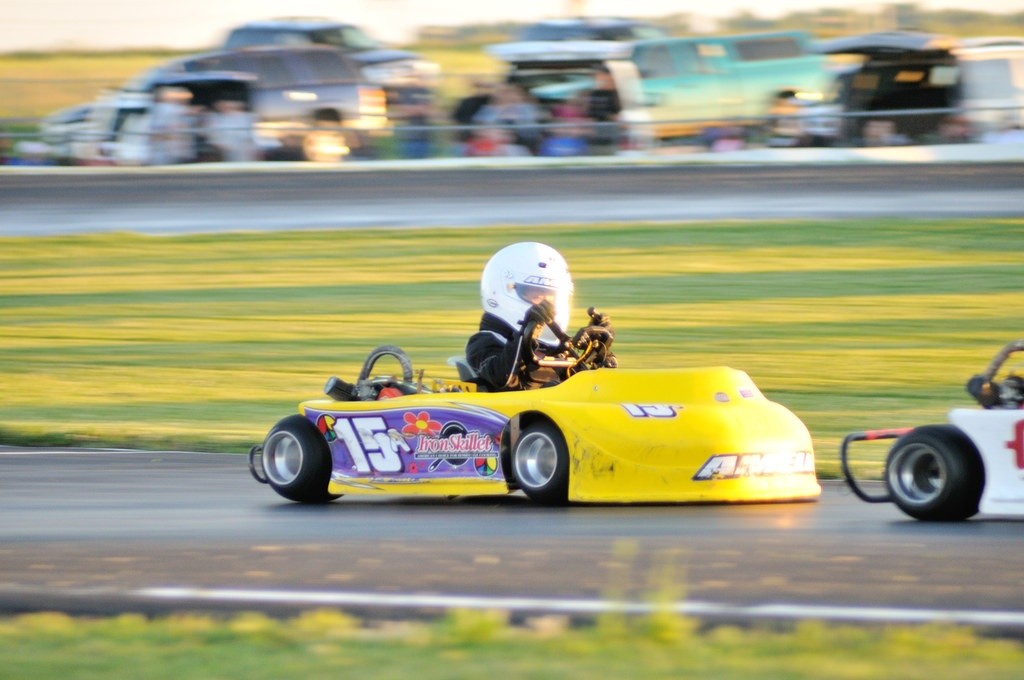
518,301,557,341
589,313,612,332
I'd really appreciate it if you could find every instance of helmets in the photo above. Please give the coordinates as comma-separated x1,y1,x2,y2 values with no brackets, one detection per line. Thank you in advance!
480,243,573,348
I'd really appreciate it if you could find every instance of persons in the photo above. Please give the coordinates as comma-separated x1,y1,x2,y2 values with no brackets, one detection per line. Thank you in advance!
467,242,616,391
141,80,812,158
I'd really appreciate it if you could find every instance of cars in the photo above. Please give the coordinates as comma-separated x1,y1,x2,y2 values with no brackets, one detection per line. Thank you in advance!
485,15,1024,156
41,16,436,163
